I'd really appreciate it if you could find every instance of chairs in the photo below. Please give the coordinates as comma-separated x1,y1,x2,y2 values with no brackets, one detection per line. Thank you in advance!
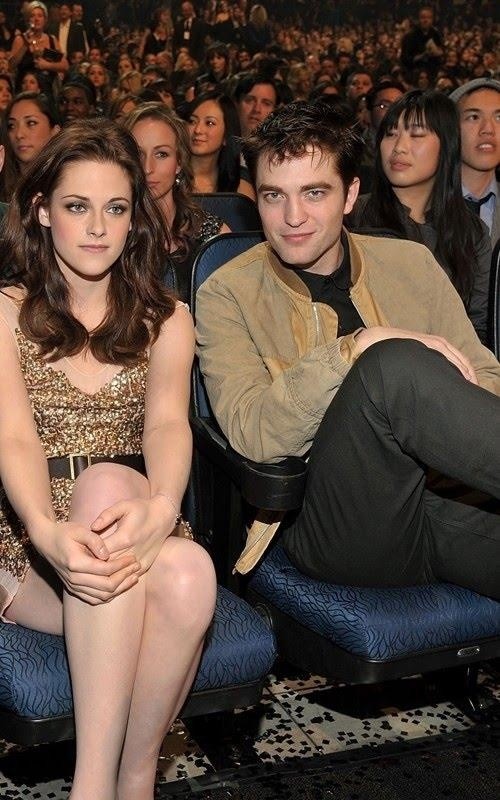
187,191,263,229
348,165,378,193
0,244,282,787
189,227,500,726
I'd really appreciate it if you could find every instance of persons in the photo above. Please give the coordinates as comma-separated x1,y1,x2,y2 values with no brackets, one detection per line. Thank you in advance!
0,1,499,352
192,100,499,607
1,117,219,799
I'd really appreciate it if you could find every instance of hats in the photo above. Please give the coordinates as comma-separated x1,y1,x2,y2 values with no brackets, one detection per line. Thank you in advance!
449,78,499,103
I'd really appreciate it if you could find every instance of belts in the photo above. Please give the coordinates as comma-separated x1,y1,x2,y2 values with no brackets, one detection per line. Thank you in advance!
48,454,148,480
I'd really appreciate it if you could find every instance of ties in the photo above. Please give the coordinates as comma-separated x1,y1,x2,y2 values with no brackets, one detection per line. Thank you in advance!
184,21,190,47
